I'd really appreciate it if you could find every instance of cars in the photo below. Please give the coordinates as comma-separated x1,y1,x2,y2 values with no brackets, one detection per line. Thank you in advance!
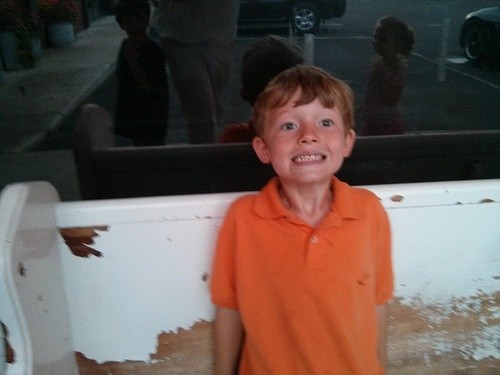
115,0,348,37
458,6,500,66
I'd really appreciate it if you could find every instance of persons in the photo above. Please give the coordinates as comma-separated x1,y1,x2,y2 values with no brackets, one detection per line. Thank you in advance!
217,35,308,144
148,0,240,144
359,15,415,137
112,0,170,149
211,64,394,375
